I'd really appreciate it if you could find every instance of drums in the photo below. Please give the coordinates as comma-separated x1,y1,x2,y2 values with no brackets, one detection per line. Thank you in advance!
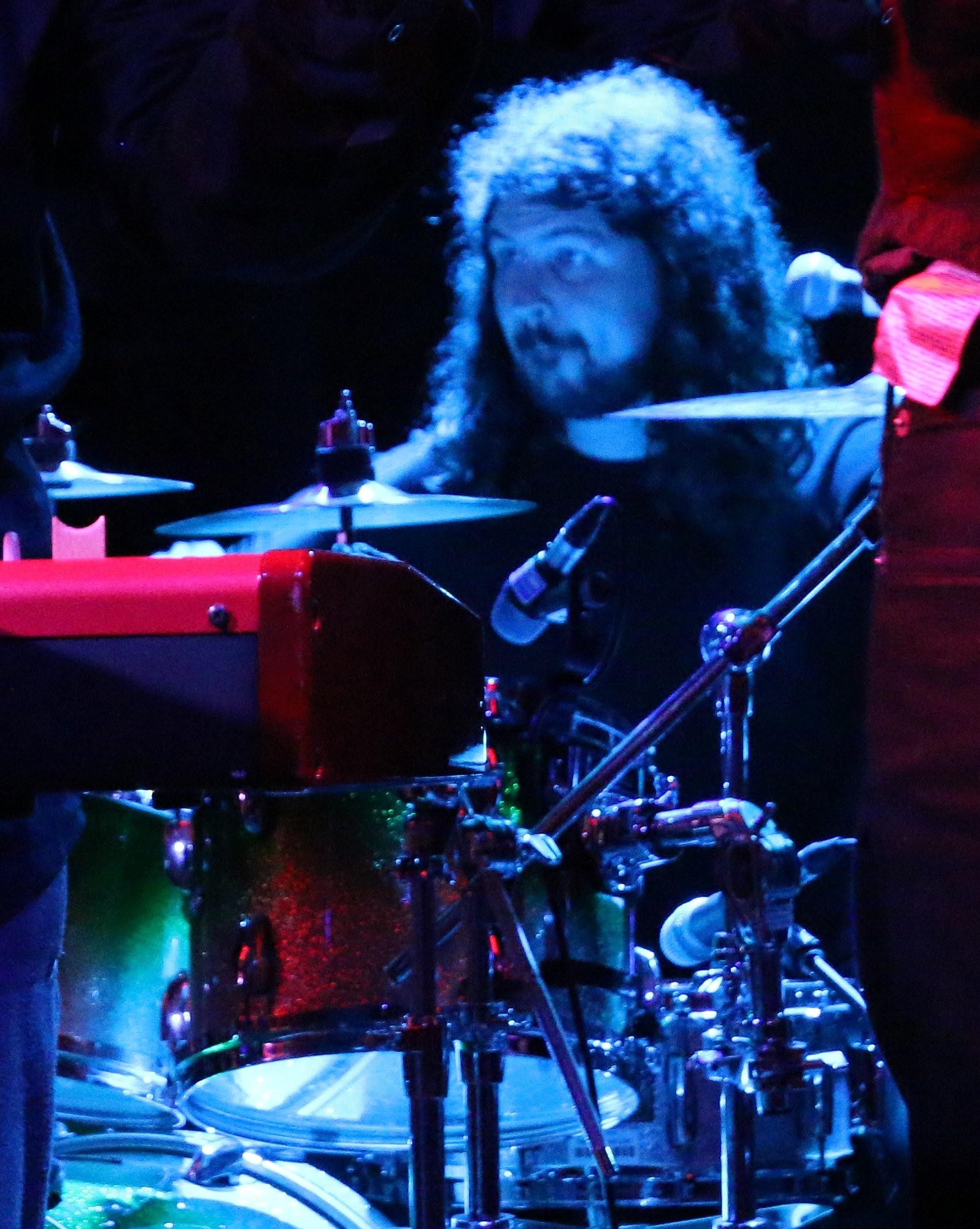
48,799,192,1128
36,1131,404,1229
447,977,877,1212
166,711,673,1155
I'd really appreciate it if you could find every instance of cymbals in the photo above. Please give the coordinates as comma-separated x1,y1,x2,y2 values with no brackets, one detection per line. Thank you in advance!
157,479,538,540
603,375,912,424
31,460,195,503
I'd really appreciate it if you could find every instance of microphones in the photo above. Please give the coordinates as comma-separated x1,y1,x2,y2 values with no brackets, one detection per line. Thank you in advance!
778,248,883,326
486,491,619,648
657,834,863,972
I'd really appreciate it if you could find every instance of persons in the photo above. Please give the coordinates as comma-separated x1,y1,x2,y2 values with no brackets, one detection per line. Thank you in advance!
157,59,880,1010
